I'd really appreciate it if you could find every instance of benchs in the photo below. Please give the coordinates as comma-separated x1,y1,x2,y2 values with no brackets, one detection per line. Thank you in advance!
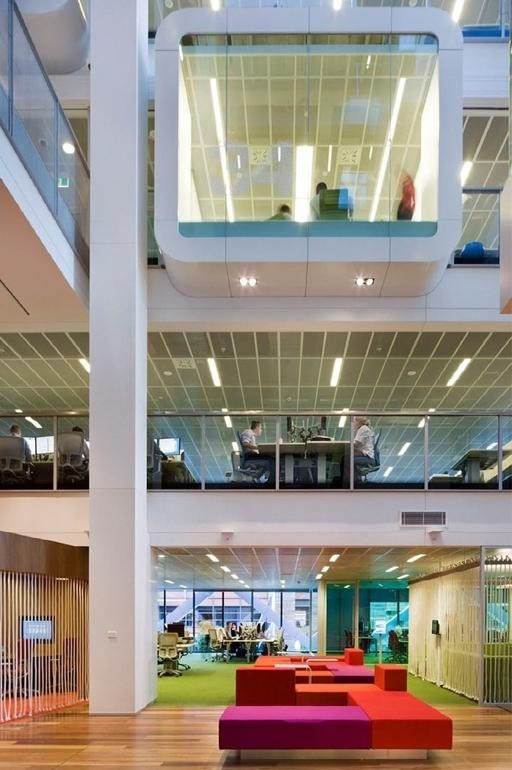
219,646,453,757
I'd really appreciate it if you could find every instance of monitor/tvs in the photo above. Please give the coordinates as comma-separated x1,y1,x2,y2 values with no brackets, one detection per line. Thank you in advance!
154,436,181,456
23,620,52,640
22,435,89,457
432,619,438,634
167,624,184,637
287,416,327,433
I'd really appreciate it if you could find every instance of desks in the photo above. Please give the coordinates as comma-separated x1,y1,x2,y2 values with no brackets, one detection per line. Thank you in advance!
358,637,373,653
429,448,512,483
257,439,354,481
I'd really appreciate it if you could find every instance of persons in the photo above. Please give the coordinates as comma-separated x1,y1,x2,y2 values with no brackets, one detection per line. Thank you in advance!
1,424,30,478
239,420,274,484
394,619,404,639
268,204,291,220
225,620,264,658
342,415,375,482
62,425,89,484
309,182,327,220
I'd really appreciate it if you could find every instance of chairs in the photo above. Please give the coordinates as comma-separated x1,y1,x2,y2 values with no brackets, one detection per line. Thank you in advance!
317,185,349,222
204,626,285,662
343,629,352,648
0,431,91,489
230,429,265,483
157,632,191,676
146,425,161,489
384,629,408,664
0,657,79,700
355,426,383,485
